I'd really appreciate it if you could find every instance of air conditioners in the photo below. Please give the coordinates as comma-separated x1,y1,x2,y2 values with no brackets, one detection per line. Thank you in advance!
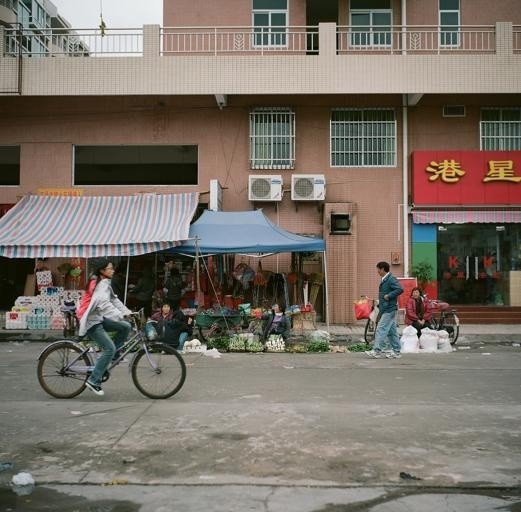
247,173,326,204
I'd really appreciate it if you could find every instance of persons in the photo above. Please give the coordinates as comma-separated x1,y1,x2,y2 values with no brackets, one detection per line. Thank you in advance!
404,287,434,338
75,258,134,396
365,261,404,359
158,311,193,349
259,301,291,341
149,303,175,321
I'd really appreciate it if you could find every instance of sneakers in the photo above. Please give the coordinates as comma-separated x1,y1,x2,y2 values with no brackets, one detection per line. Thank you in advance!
386,353,401,359
365,350,381,358
112,356,125,362
86,381,104,395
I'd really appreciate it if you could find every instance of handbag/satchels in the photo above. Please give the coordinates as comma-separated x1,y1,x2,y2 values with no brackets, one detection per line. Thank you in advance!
355,304,371,319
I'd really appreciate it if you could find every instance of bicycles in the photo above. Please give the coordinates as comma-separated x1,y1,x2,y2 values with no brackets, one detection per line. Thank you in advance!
422,294,459,345
360,295,380,344
37,309,185,399
